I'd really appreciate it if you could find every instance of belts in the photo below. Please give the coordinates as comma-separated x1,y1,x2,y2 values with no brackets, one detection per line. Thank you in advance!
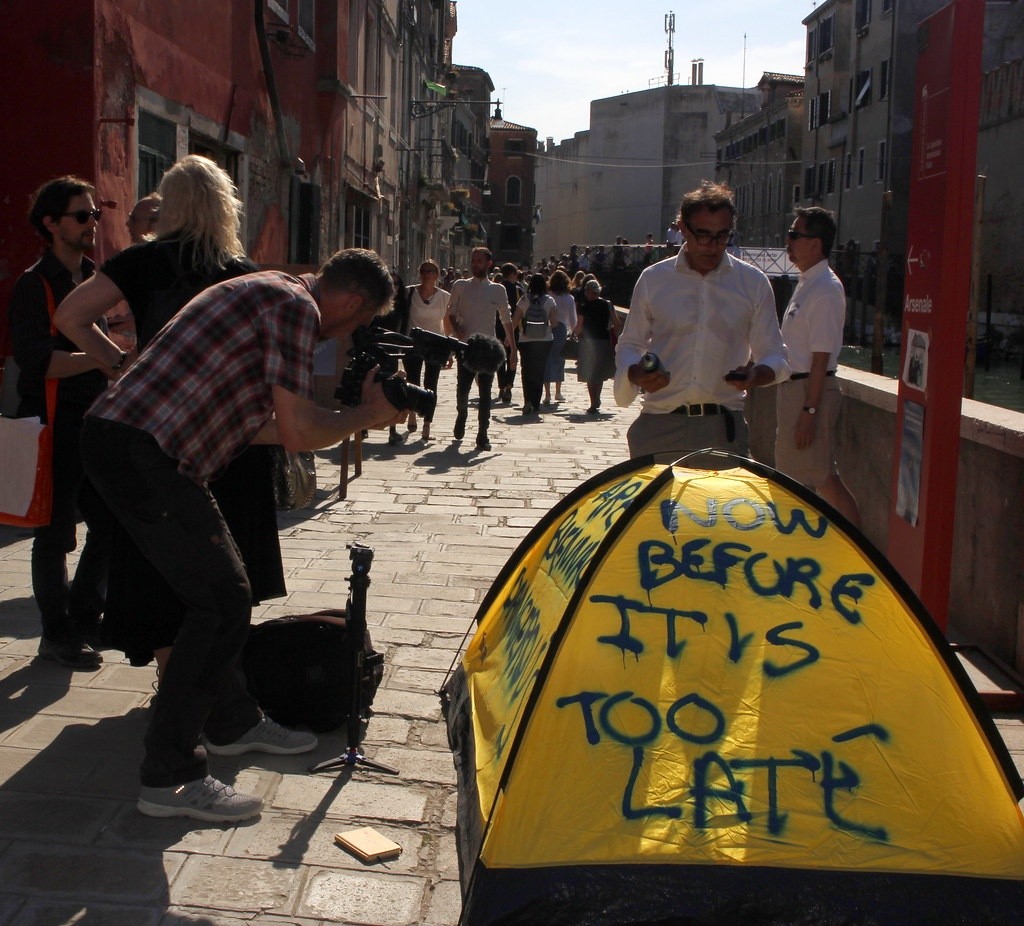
673,402,735,443
789,372,833,381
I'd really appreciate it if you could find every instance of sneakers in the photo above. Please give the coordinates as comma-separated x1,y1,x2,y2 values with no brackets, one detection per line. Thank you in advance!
138,775,264,821
202,716,319,756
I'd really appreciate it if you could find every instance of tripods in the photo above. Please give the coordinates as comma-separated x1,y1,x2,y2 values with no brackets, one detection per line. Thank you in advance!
308,544,399,775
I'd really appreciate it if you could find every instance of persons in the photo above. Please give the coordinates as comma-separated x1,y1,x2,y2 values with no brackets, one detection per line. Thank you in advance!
727,231,741,258
81,247,407,824
595,222,682,270
362,272,409,444
438,245,592,364
447,246,517,448
8,175,108,666
613,182,791,459
496,263,524,401
125,194,160,243
48,154,286,744
405,260,454,440
774,207,859,527
543,269,577,404
574,280,620,412
505,272,557,414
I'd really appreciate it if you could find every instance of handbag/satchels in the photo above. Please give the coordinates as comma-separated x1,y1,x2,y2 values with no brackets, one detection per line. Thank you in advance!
0,416,54,528
564,333,580,359
607,302,619,351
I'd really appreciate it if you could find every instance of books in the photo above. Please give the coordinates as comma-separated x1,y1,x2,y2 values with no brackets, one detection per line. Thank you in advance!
335,826,400,862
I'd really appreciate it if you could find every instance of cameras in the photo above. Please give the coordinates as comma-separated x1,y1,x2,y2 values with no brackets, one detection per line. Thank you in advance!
336,325,454,418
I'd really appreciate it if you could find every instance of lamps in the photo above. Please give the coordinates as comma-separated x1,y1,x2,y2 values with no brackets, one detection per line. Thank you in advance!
409,99,505,126
422,177,491,195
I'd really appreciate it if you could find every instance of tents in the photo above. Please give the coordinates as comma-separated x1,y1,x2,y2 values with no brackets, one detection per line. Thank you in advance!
443,448,1023,925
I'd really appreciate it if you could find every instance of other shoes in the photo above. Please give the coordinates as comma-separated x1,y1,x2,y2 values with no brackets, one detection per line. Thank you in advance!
476,436,492,451
543,395,550,403
362,429,369,439
587,400,600,412
407,415,417,432
523,403,539,413
389,432,403,444
422,425,430,441
38,625,103,667
500,387,511,404
454,413,467,439
555,394,565,401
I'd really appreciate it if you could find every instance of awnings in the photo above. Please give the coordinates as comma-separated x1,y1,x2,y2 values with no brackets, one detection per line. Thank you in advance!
424,80,446,96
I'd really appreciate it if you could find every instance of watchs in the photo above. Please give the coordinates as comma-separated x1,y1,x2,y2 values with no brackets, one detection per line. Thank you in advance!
803,406,817,414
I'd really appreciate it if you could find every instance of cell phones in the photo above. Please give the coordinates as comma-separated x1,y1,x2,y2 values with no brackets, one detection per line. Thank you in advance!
725,372,746,381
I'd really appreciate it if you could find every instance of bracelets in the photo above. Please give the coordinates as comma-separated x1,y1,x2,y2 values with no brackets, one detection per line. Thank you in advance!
111,352,129,370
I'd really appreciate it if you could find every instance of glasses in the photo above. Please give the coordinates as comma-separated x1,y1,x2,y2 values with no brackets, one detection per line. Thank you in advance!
788,229,821,240
687,224,733,245
129,212,159,226
53,209,103,225
419,270,437,275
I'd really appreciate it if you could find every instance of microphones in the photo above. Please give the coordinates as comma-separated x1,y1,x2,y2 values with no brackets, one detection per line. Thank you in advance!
455,334,507,375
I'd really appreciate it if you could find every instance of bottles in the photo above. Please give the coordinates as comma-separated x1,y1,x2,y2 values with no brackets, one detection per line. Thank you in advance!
639,352,671,386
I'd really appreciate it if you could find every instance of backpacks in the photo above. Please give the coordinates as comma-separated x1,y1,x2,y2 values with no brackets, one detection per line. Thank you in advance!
522,294,547,338
